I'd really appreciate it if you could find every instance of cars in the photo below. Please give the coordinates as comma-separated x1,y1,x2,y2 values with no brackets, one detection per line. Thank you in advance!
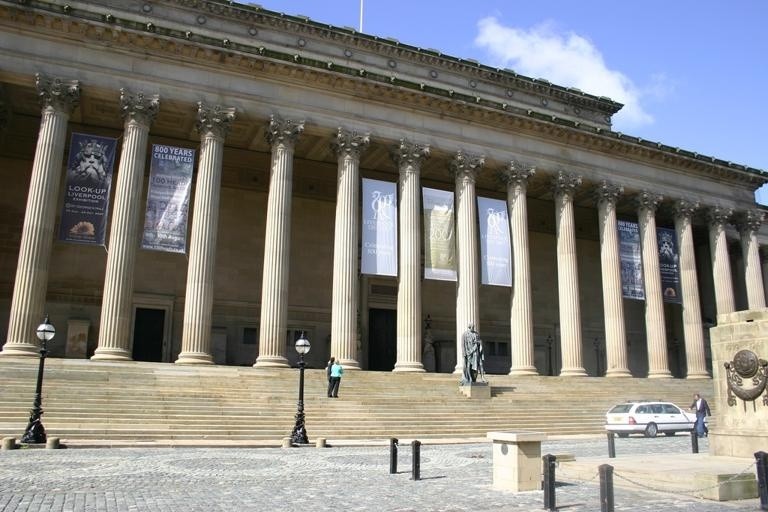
605,399,709,438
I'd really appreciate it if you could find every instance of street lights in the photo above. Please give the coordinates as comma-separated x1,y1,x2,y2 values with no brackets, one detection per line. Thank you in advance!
289,330,311,444
19,312,55,444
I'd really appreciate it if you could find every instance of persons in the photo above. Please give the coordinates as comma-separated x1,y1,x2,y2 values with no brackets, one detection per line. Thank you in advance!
658,229,677,263
688,393,712,438
459,321,482,384
326,361,343,398
67,138,112,190
326,358,334,379
